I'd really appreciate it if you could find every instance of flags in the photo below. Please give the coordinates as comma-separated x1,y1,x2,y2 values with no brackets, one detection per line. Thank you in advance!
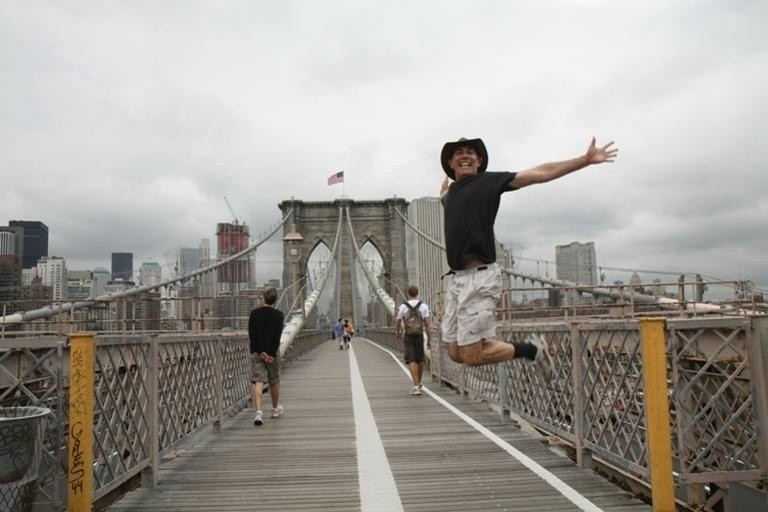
327,170,344,185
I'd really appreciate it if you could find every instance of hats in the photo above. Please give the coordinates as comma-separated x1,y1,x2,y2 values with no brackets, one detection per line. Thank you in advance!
441,137,488,180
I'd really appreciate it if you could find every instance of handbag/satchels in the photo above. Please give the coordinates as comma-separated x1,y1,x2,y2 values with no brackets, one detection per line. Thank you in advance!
346,327,352,334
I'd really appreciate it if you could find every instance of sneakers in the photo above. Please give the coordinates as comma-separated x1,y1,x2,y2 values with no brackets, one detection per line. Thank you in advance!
339,342,349,350
254,413,263,424
272,405,282,418
524,334,555,383
410,383,422,395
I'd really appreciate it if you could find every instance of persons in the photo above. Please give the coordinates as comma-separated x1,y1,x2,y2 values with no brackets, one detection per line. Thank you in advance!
395,284,434,396
437,135,622,383
329,316,362,351
243,284,285,426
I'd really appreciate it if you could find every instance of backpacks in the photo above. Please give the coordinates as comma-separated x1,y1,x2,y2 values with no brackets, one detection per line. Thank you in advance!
404,301,423,337
